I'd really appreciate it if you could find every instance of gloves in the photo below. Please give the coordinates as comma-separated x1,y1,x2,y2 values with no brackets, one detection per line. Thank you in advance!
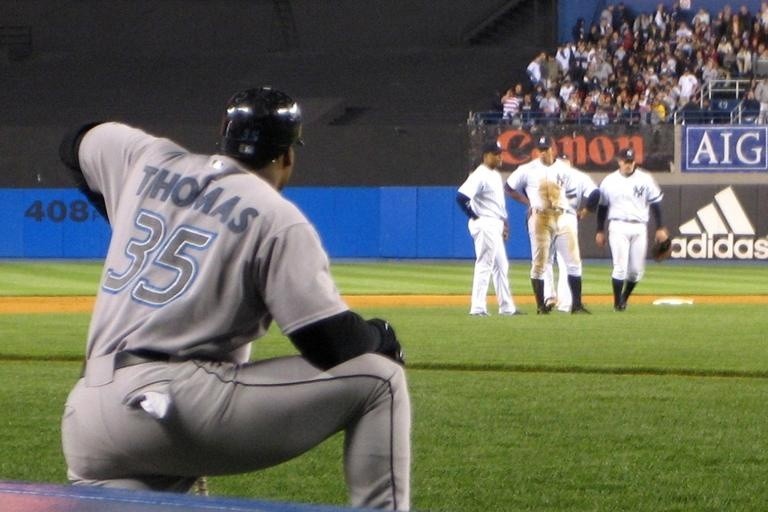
366,318,404,363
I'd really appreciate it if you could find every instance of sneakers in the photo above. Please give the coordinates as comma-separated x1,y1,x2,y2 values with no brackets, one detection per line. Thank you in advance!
500,308,527,316
544,298,556,311
469,310,491,316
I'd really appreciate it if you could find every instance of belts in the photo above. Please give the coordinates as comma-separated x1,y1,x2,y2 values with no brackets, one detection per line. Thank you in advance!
611,218,642,224
79,346,224,375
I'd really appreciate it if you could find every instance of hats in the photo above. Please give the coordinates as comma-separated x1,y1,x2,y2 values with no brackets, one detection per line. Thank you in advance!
617,148,635,162
482,142,506,155
535,134,552,148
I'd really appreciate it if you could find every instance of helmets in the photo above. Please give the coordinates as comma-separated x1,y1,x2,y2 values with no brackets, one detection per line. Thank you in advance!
219,85,306,159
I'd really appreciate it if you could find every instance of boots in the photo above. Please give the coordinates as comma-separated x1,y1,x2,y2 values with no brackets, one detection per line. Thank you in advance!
613,278,637,311
568,274,593,315
530,278,552,314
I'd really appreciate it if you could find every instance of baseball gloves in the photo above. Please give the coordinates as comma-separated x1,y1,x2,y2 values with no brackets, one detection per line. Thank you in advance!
652,238,672,262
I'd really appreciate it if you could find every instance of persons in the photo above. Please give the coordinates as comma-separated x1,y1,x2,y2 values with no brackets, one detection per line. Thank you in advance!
454,133,666,317
473,1,768,127
50,81,415,510
